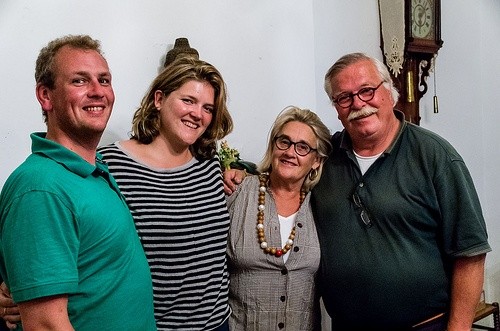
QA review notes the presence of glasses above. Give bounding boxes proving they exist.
[350,189,375,231]
[333,80,385,109]
[273,134,319,157]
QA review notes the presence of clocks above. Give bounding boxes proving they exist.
[377,0,445,126]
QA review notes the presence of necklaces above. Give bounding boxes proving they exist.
[257,175,306,258]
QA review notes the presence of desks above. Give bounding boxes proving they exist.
[471,301,500,331]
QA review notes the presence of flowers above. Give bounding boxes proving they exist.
[219,140,240,171]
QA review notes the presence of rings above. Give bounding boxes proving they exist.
[4,307,7,314]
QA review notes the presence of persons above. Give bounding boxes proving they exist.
[225,104,332,331]
[0,57,235,331]
[222,51,492,331]
[0,34,158,331]
